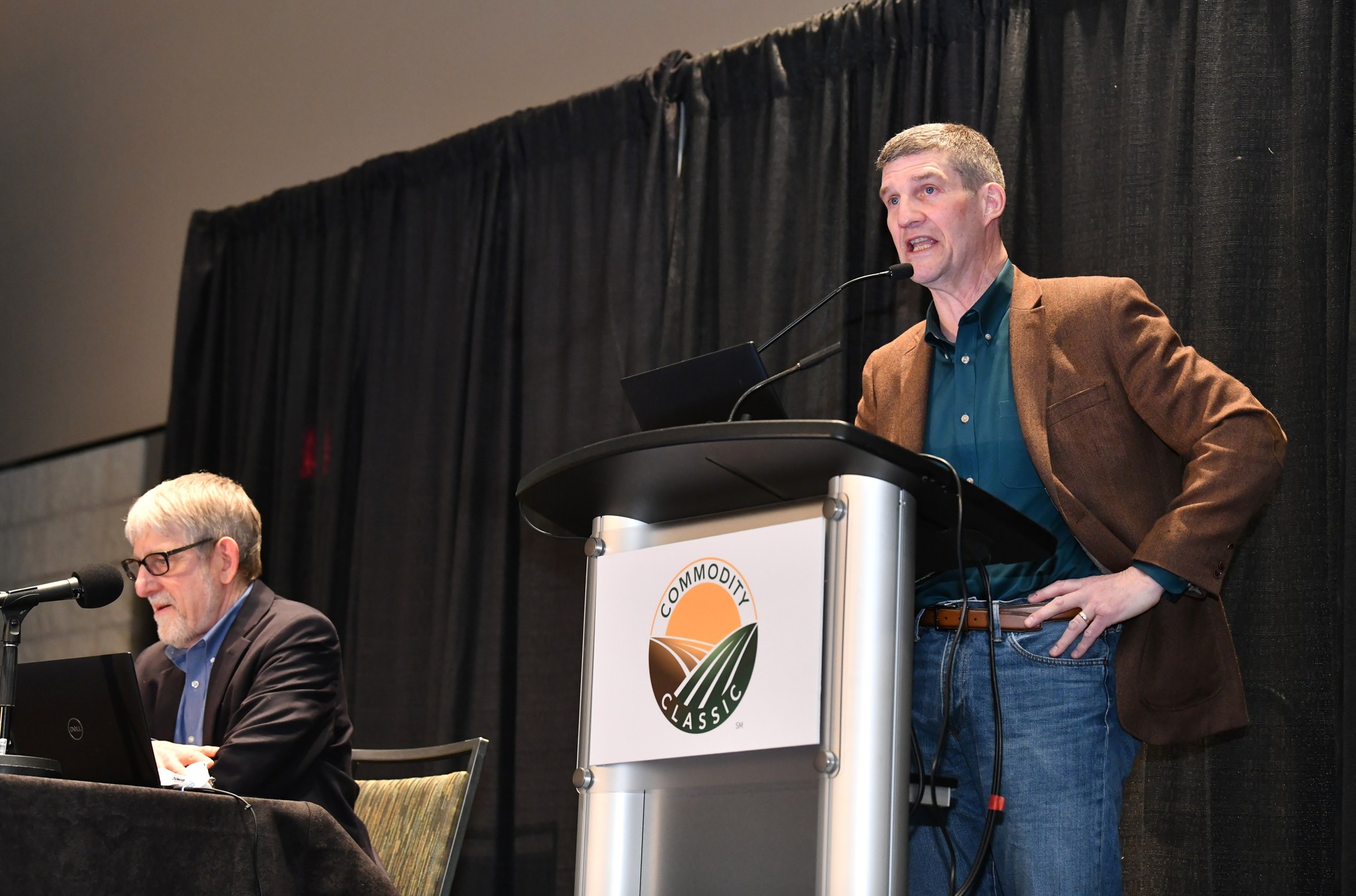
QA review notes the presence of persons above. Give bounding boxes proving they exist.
[850,117,1293,896]
[123,472,360,823]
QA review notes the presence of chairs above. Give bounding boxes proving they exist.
[343,736,491,896]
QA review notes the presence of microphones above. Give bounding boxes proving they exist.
[0,562,124,626]
[726,340,843,422]
[173,786,193,792]
[753,263,914,355]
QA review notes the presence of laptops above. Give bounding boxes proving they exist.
[6,653,162,788]
[623,342,789,429]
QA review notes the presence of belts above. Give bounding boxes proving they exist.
[920,603,1082,632]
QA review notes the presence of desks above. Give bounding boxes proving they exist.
[0,767,390,896]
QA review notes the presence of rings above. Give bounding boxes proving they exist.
[1078,612,1091,624]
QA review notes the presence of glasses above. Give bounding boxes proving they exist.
[120,538,212,581]
